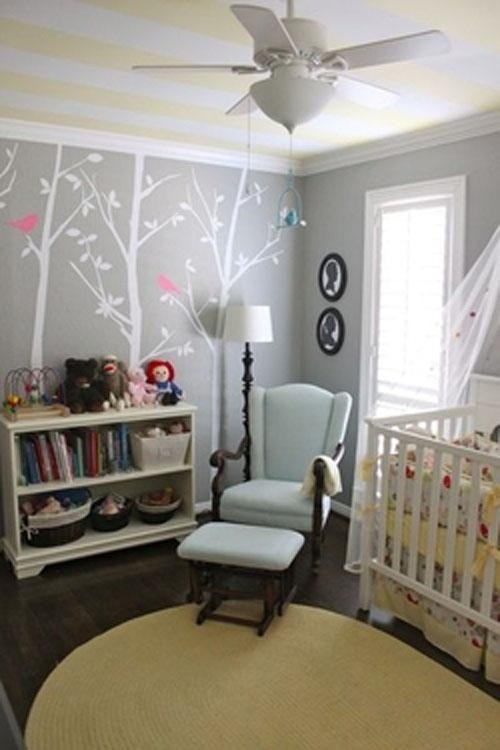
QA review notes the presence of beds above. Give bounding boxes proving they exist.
[357,372,500,690]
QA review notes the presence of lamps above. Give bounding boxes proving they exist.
[249,66,335,231]
[221,305,275,480]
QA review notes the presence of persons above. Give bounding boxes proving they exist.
[321,315,336,346]
[324,262,338,294]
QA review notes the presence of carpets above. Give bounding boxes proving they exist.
[22,595,499,750]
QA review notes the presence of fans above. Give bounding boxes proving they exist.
[129,1,453,119]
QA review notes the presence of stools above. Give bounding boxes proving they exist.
[176,520,306,636]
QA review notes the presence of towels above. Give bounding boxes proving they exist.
[299,454,343,500]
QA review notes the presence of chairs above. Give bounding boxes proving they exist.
[208,382,353,577]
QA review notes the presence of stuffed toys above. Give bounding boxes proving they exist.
[145,419,183,437]
[147,359,186,405]
[100,353,132,410]
[126,366,157,408]
[57,358,109,412]
[22,491,84,515]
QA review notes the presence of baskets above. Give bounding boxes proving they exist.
[90,496,133,531]
[137,488,180,522]
[18,488,91,547]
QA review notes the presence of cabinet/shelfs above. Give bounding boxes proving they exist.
[1,401,197,579]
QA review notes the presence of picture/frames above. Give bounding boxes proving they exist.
[316,306,344,356]
[318,253,348,303]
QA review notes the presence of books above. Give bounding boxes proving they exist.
[15,423,129,485]
